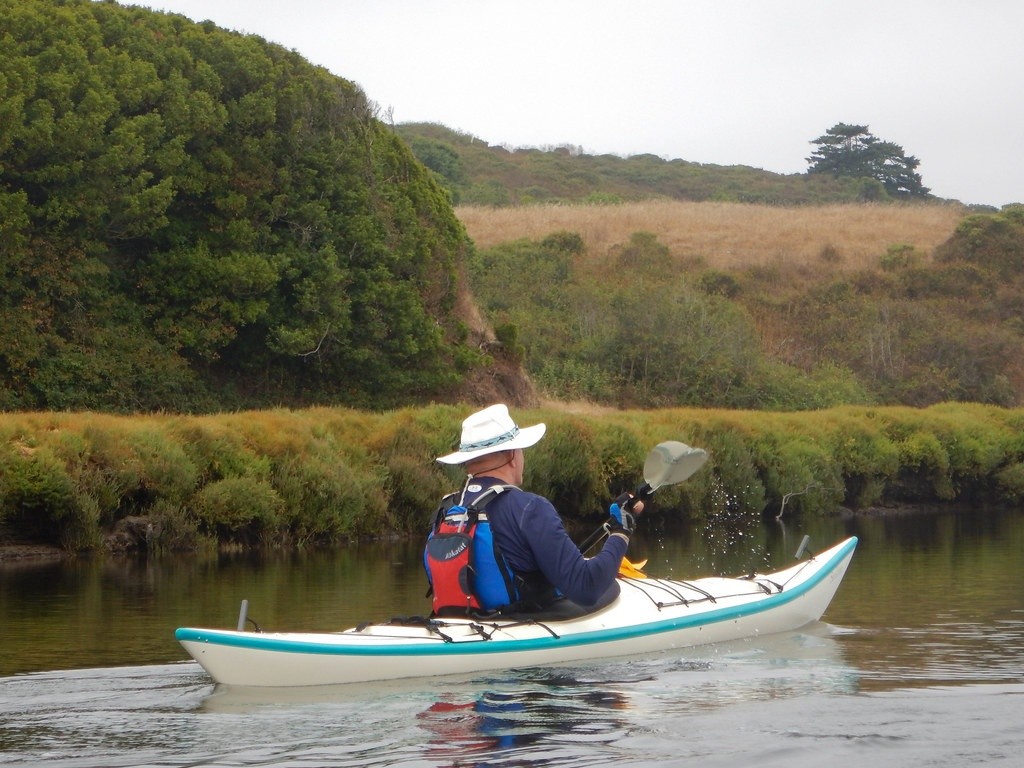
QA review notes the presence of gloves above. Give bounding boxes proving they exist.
[604,492,645,539]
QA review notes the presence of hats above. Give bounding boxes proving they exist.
[436,403,546,465]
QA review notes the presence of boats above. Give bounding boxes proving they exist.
[174,536,859,686]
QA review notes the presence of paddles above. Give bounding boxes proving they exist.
[578,440,708,555]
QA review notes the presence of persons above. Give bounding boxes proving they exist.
[423,403,639,616]
